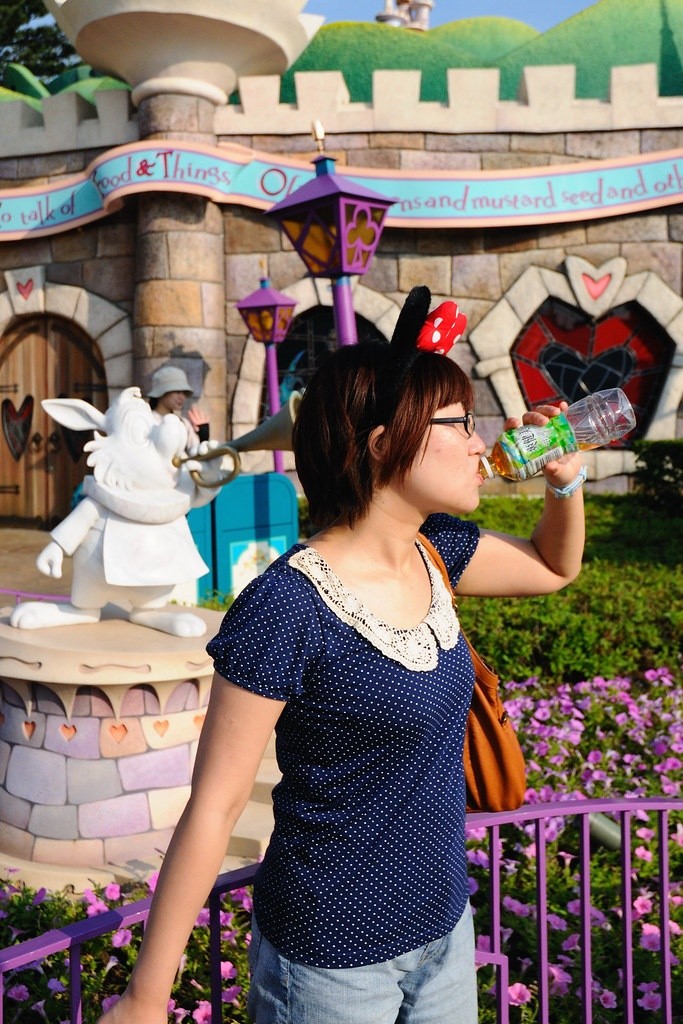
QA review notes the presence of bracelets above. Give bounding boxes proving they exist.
[544,467,586,499]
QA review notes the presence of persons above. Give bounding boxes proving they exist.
[98,343,586,1024]
[147,365,201,456]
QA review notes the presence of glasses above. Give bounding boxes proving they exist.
[428,412,475,436]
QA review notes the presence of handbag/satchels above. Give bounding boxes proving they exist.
[416,532,527,812]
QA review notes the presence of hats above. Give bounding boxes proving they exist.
[146,367,193,399]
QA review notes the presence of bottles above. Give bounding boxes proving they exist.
[478,386,637,479]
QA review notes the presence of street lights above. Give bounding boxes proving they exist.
[233,258,303,475]
[265,118,402,344]
